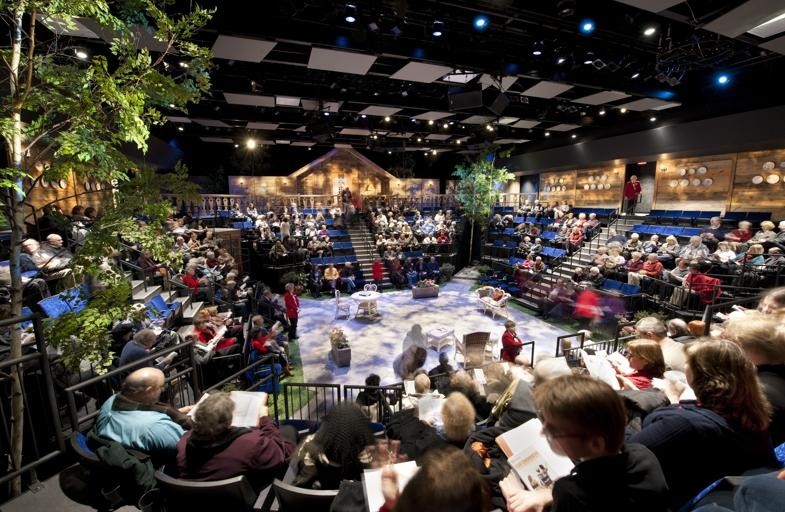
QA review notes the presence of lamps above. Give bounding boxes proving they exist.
[431,17,445,38]
[342,0,359,25]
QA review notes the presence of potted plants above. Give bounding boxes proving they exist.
[279,270,311,296]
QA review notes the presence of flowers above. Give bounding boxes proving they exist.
[328,326,349,349]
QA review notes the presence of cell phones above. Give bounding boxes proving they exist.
[773,441,785,466]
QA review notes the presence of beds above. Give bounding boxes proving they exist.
[425,326,455,353]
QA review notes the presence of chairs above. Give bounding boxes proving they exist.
[593,209,773,315]
[247,344,282,393]
[452,331,491,371]
[0,238,181,403]
[217,207,366,290]
[402,204,615,298]
[333,281,382,321]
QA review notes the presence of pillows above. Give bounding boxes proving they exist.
[478,289,488,299]
[490,289,503,301]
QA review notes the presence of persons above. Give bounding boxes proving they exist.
[19,189,785,512]
[623,175,642,214]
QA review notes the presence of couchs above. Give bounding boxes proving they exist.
[473,284,511,321]
